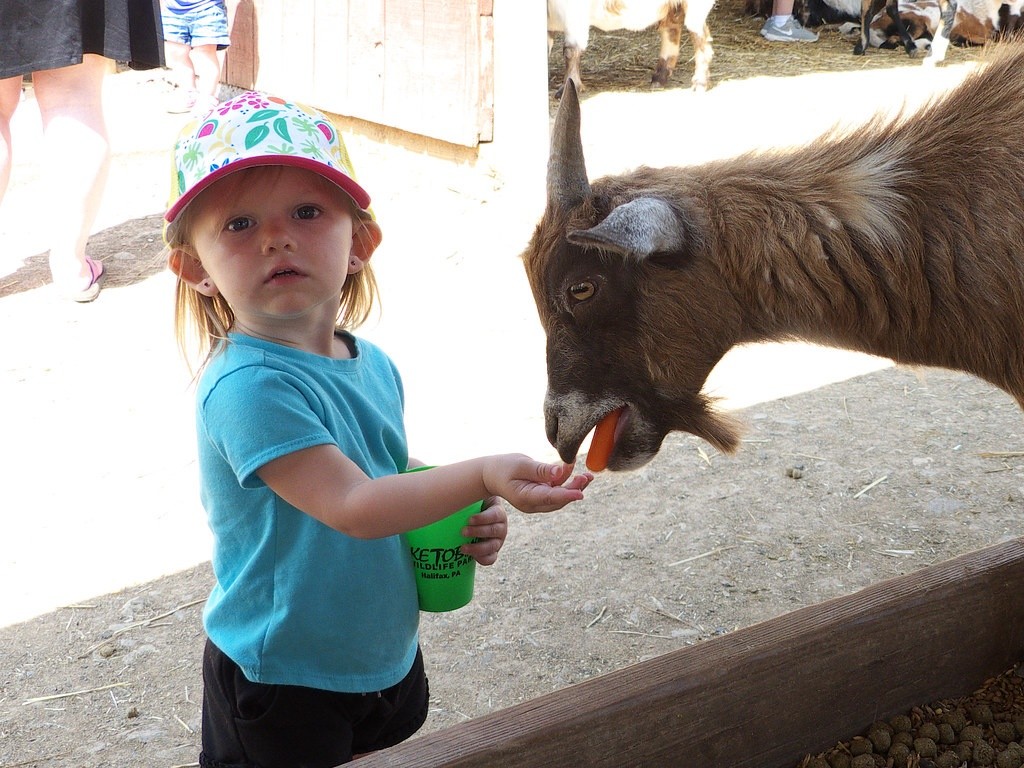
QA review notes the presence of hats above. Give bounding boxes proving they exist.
[165,95,369,231]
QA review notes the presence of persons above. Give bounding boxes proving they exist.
[160,92,593,766]
[0,1,166,303]
[165,1,233,114]
[760,0,820,43]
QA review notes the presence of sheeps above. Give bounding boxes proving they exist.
[519,28,1024,472]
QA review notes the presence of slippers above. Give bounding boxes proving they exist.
[69,256,107,302]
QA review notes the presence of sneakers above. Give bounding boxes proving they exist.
[760,17,772,37]
[764,16,818,43]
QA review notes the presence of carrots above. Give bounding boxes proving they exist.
[586,408,622,472]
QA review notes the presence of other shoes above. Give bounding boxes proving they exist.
[194,96,221,122]
[167,99,194,115]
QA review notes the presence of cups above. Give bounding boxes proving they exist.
[401,465,484,613]
